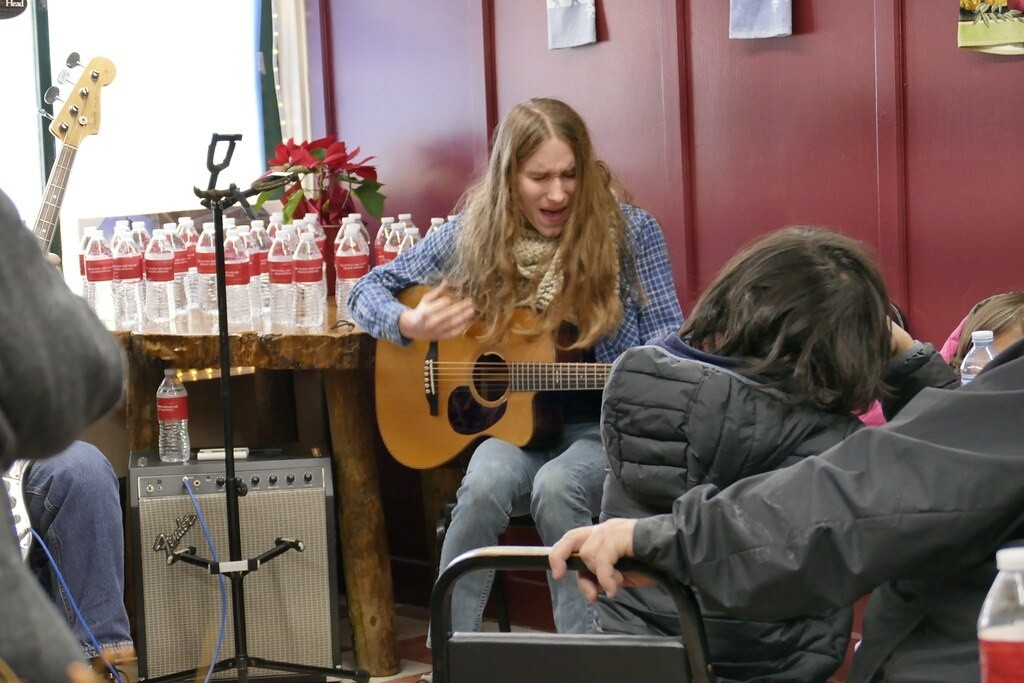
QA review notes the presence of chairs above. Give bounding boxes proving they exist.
[433,488,714,683]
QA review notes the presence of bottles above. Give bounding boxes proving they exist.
[155,368,191,463]
[977,547,1024,683]
[960,330,999,388]
[79,211,458,336]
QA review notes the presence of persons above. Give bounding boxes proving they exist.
[849,292,1024,430]
[547,339,1024,683]
[348,97,684,682]
[599,221,963,683]
[0,187,142,683]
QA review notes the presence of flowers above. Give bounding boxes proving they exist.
[267,133,388,226]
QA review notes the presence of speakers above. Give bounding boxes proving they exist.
[128,441,340,683]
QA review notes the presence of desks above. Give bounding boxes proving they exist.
[111,297,414,678]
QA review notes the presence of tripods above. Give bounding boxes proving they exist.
[133,186,370,683]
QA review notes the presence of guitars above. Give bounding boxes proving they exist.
[0,48,121,562]
[368,279,617,476]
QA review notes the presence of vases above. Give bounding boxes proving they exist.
[322,225,341,293]
